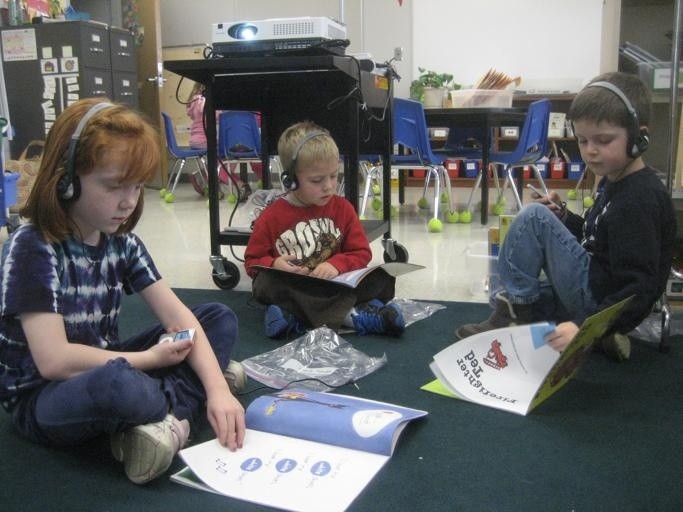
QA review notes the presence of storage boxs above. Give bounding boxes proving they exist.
[450,90,513,108]
[636,60,683,94]
[413,158,585,180]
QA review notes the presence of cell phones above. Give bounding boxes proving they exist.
[156,328,196,343]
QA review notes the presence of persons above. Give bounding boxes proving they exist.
[243,121,405,339]
[215,110,273,203]
[453,72,677,361]
[0,97,252,483]
[184,81,222,194]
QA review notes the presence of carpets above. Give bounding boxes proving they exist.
[0,286,683,511]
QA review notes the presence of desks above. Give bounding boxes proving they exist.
[396,106,527,225]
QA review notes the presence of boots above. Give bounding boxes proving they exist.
[453,293,535,342]
[600,330,632,364]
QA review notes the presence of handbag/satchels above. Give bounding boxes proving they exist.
[4,139,46,214]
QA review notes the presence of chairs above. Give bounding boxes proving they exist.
[359,99,460,233]
[218,111,263,203]
[417,122,503,222]
[459,98,550,224]
[159,112,208,203]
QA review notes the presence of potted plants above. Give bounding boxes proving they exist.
[408,66,458,108]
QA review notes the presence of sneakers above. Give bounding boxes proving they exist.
[238,182,251,203]
[108,413,191,488]
[348,298,409,335]
[216,359,249,391]
[261,304,304,337]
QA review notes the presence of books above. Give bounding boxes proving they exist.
[247,261,426,288]
[420,292,636,414]
[616,39,673,74]
[170,385,432,511]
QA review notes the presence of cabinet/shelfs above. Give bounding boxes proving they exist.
[601,0,683,195]
[2,20,138,163]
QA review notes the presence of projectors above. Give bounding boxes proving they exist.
[210,15,348,55]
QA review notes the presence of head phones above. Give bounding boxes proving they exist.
[281,132,329,190]
[56,102,115,202]
[570,80,651,158]
